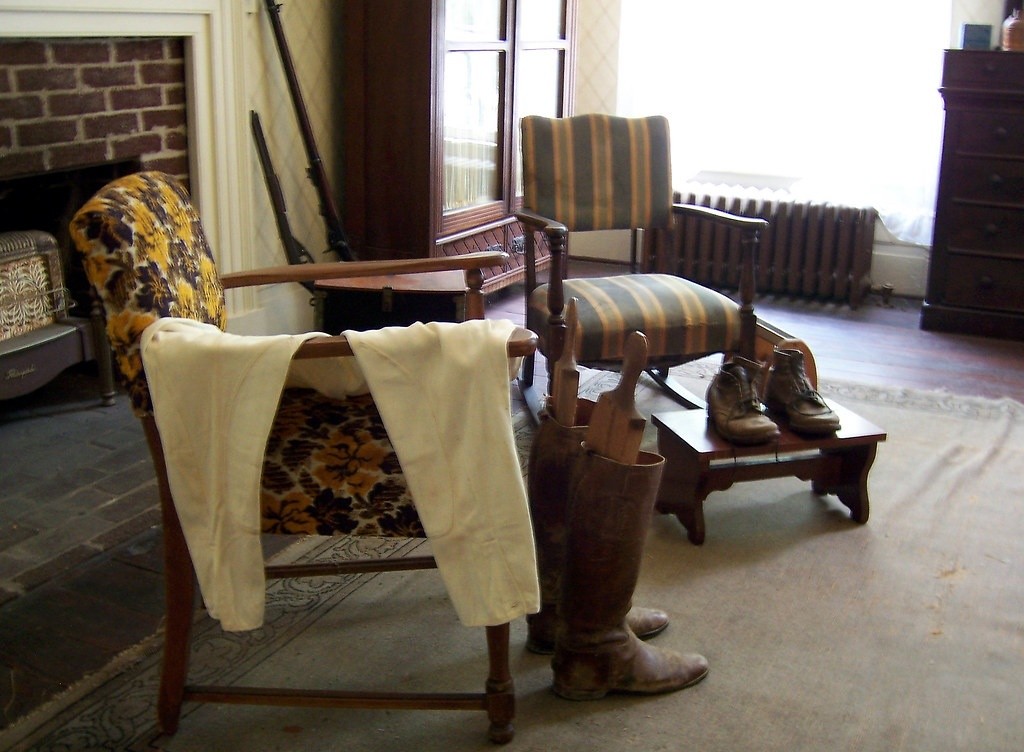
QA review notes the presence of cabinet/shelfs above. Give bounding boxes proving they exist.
[343,0,577,296]
[920,49,1024,343]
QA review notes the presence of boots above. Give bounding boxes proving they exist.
[528,398,670,655]
[707,356,781,443]
[551,441,709,700]
[761,349,842,434]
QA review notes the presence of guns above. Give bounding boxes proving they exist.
[263,0,359,263]
[248,107,320,297]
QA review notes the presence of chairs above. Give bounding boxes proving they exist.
[0,172,539,752]
[514,113,771,425]
[0,230,118,407]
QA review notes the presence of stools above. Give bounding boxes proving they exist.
[651,398,887,545]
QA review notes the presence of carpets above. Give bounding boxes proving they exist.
[145,362,1024,752]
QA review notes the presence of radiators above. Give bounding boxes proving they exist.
[641,192,878,311]
